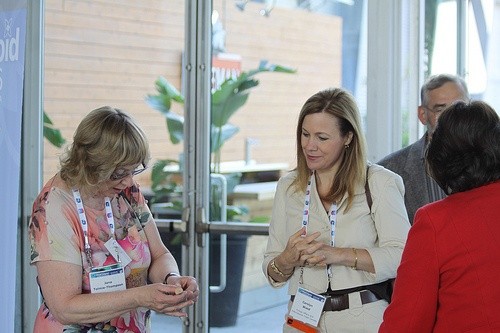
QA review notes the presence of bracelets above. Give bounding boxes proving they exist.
[163,273,179,284]
[351,247,359,270]
[271,258,294,279]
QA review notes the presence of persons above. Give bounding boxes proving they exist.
[29,106,200,333]
[373,74,469,226]
[378,101,500,333]
[262,86,413,333]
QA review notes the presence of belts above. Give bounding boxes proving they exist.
[290,289,380,312]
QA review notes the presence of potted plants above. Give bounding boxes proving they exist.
[144,61,297,328]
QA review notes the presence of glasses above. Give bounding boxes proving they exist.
[110,160,147,180]
[424,105,444,118]
